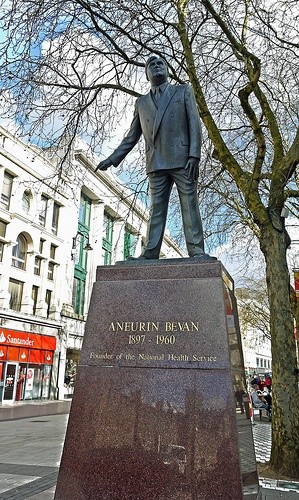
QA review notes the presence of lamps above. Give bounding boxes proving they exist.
[71,231,93,260]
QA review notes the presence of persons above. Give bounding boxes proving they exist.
[251,372,273,421]
[93,56,212,260]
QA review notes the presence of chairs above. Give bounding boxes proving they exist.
[250,395,271,422]
